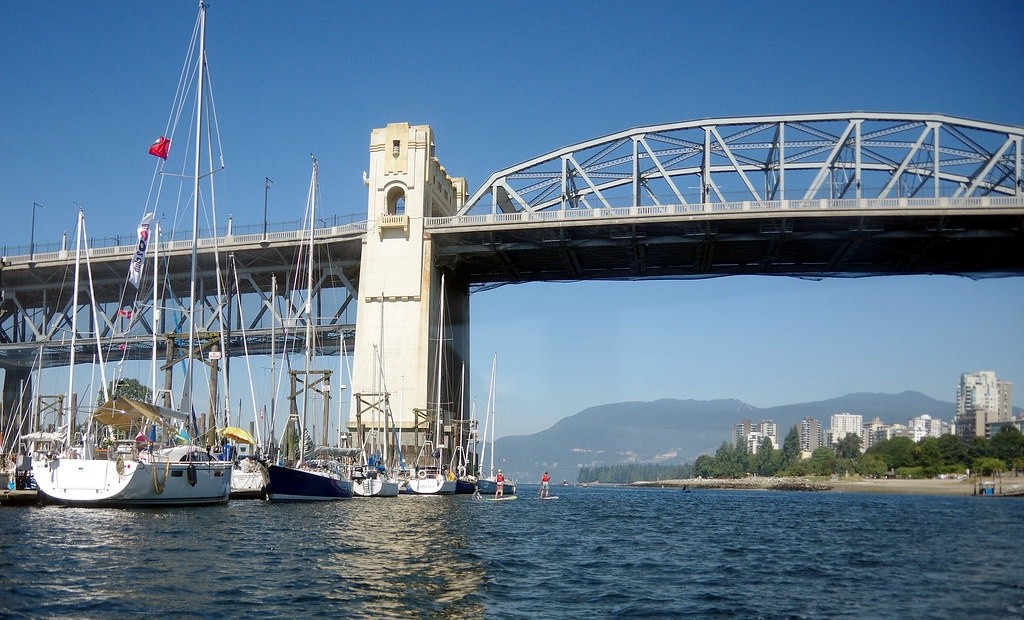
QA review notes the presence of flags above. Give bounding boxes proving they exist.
[149,136,171,160]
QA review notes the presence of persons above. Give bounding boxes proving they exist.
[540,470,550,499]
[144,441,155,454]
[495,469,505,498]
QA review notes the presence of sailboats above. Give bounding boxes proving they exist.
[1,1,518,508]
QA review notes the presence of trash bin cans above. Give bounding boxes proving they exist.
[984,481,995,495]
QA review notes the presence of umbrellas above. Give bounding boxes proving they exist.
[215,427,255,461]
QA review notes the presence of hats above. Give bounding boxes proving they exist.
[545,471,548,474]
[498,469,502,473]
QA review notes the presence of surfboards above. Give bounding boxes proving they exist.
[534,496,558,499]
[486,495,517,501]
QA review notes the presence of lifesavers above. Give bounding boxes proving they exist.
[418,470,427,479]
